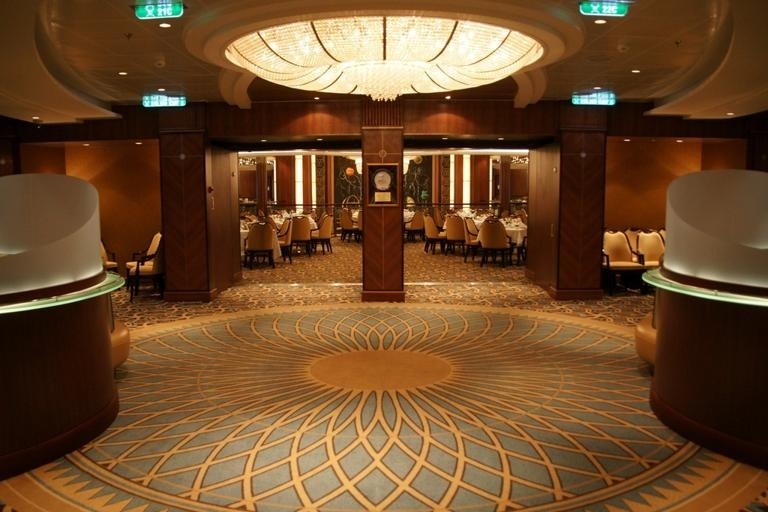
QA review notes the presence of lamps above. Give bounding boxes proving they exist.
[219,9,549,103]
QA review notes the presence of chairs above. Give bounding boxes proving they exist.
[463,217,488,264]
[126,231,162,291]
[404,212,424,241]
[659,229,667,242]
[423,215,445,255]
[445,214,466,256]
[433,207,444,232]
[603,230,643,296]
[101,242,121,292]
[341,212,359,243]
[128,244,165,303]
[516,236,528,266]
[625,230,641,262]
[478,217,512,268]
[277,220,293,265]
[290,216,312,257]
[357,211,364,243]
[244,222,276,271]
[309,216,333,256]
[637,232,667,296]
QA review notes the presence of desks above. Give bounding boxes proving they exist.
[1,173,125,483]
[641,170,768,472]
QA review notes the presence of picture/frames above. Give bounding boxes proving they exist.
[366,163,400,208]
